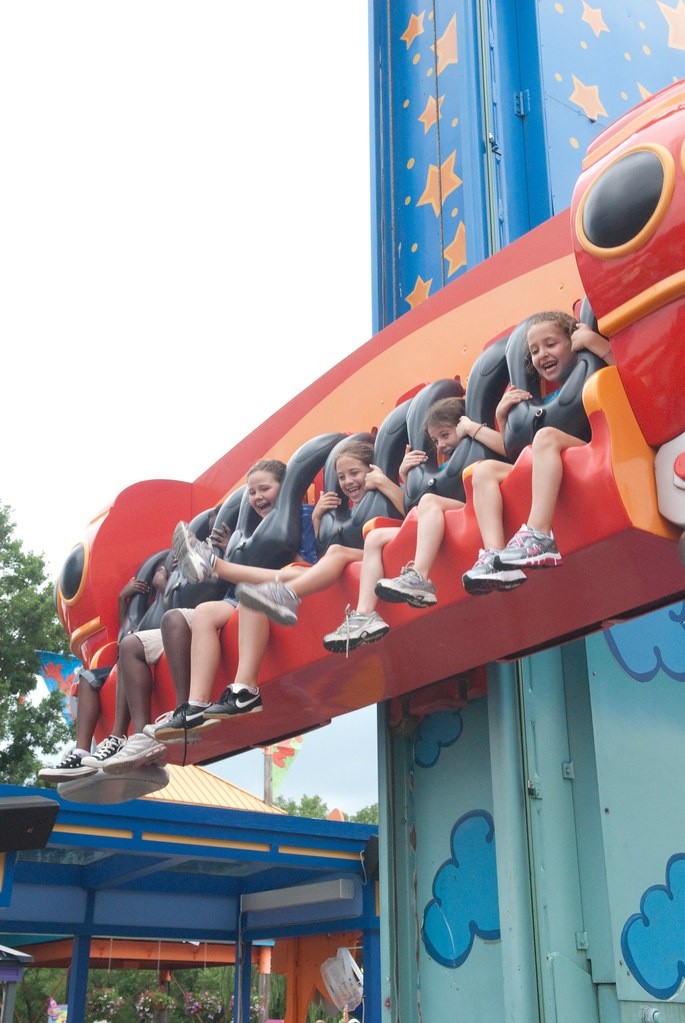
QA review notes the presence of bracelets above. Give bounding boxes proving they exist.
[472,424,487,440]
[599,349,611,358]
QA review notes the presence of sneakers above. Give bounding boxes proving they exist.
[101,732,168,775]
[81,735,126,769]
[203,686,263,719]
[321,603,389,658]
[234,576,300,625]
[172,520,219,584]
[143,710,182,743]
[155,702,221,767]
[492,523,563,570]
[374,560,437,609]
[462,547,526,595]
[38,753,99,783]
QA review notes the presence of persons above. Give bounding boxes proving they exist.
[101,504,233,774]
[37,566,168,783]
[462,311,617,596]
[154,459,317,767]
[322,396,506,659]
[173,440,404,627]
